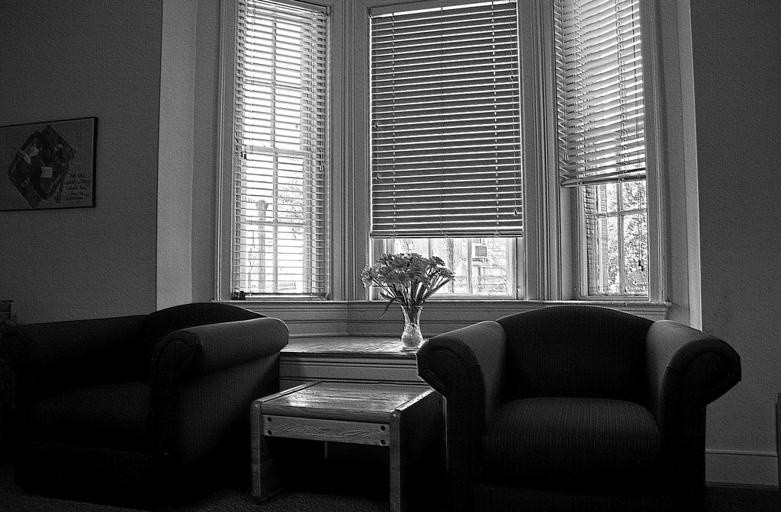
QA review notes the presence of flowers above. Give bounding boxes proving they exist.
[361,253,455,324]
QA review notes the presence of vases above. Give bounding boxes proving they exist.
[400,306,423,350]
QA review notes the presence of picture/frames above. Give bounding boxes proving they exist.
[0,117,97,212]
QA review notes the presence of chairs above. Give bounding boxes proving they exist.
[417,305,741,511]
[11,302,290,511]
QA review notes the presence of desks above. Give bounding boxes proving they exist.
[249,380,444,511]
